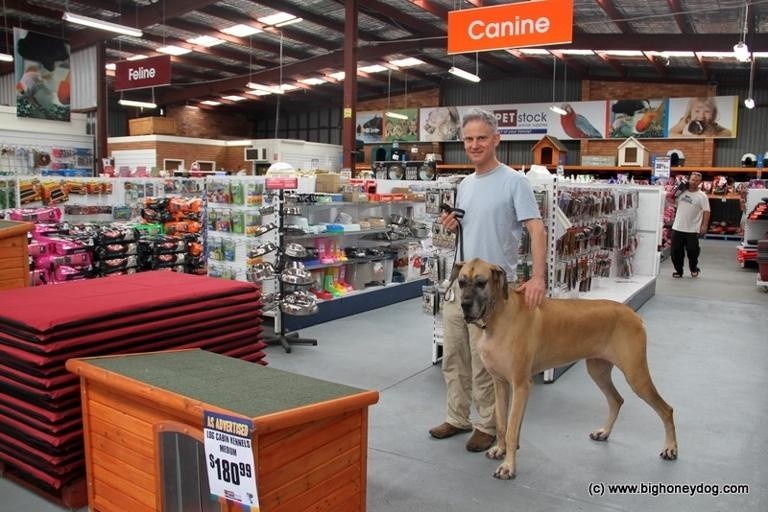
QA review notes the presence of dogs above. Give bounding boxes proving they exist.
[449,257,677,480]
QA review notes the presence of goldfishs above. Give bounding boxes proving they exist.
[636,98,663,132]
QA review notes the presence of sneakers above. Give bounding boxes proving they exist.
[467,428,496,452]
[673,270,681,278]
[691,271,698,277]
[429,422,472,438]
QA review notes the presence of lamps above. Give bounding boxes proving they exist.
[118,35,158,110]
[385,68,408,121]
[734,1,749,63]
[448,1,480,84]
[550,55,569,115]
[62,1,144,39]
[743,51,758,110]
[246,34,285,95]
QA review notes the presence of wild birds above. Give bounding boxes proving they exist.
[559,102,602,138]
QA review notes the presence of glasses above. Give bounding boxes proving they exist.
[692,172,701,177]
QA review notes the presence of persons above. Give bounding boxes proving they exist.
[667,96,732,138]
[384,115,419,138]
[668,170,711,278]
[17,66,53,100]
[423,105,549,453]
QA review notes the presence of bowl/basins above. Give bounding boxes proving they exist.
[246,194,320,317]
[388,213,408,241]
